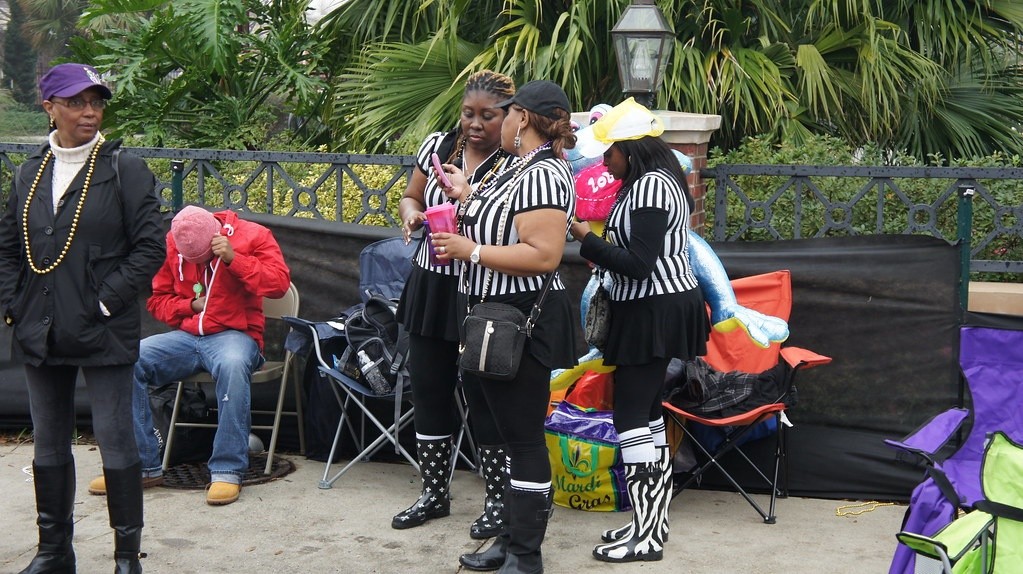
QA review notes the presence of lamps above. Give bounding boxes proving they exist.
[609,0,679,109]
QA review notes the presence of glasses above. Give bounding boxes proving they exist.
[48,98,107,111]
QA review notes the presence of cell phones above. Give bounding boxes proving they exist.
[432,152,453,188]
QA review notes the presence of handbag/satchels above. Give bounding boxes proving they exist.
[585,285,611,355]
[459,302,526,380]
[543,398,633,512]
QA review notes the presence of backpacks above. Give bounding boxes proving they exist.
[338,288,411,393]
[148,382,211,465]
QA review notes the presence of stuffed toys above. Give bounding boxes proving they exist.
[548,103,789,391]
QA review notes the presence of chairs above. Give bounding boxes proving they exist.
[160,276,300,477]
[661,270,831,525]
[282,235,483,493]
[885,312,1023,574]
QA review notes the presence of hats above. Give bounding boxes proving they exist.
[38,63,112,101]
[494,80,571,121]
[573,96,664,159]
[171,205,221,264]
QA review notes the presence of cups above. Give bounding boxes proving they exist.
[424,202,460,265]
[423,220,452,267]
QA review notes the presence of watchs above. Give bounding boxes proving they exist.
[470,245,481,264]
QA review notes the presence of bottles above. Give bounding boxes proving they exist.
[357,350,392,395]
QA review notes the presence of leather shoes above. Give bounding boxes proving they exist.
[204,482,242,505]
[88,474,163,495]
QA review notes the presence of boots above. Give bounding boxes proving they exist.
[496,484,554,574]
[102,460,147,574]
[602,445,673,542]
[592,459,665,562]
[18,458,76,574]
[458,472,510,570]
[470,444,506,539]
[391,435,452,530]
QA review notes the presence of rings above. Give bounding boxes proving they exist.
[402,228,404,231]
[440,247,445,254]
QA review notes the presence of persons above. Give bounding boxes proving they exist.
[391,71,515,539]
[429,81,574,574]
[570,96,710,563]
[88,206,289,505]
[0,64,167,574]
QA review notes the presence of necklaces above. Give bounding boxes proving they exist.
[458,138,551,303]
[192,264,207,299]
[22,138,103,274]
[836,501,895,516]
[602,186,626,239]
